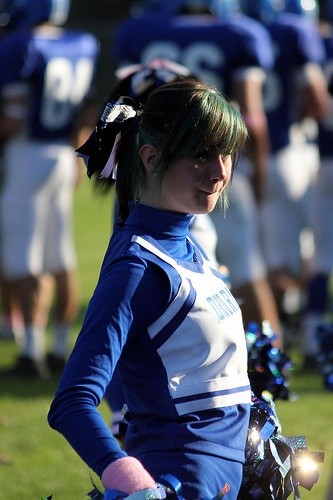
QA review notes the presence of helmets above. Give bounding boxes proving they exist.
[7,0,70,27]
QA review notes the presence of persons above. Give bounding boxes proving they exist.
[0,0,333,399]
[47,59,254,500]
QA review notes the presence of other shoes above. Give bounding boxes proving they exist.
[46,354,64,373]
[15,355,38,380]
